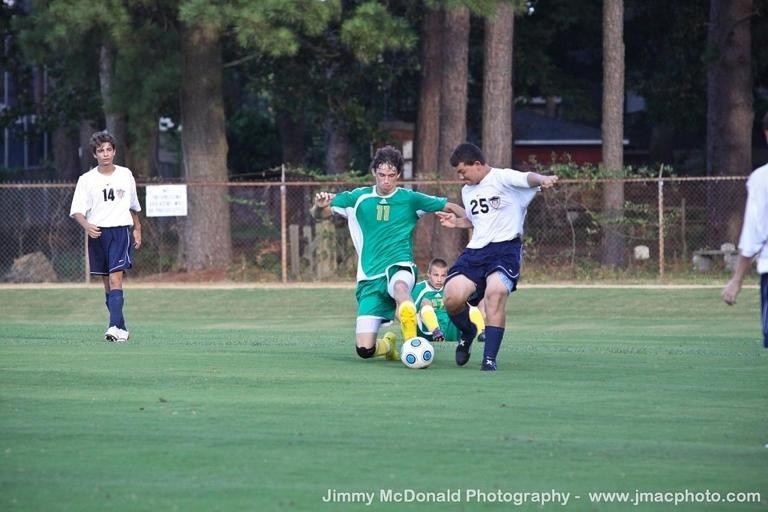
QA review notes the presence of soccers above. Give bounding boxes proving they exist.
[399,336,434,369]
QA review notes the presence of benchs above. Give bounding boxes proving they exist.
[693,251,739,274]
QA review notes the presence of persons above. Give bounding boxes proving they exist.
[310,144,474,362]
[434,142,559,372]
[721,113,768,349]
[69,131,142,342]
[410,258,487,342]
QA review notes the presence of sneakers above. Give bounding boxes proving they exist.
[454,326,477,367]
[116,329,129,342]
[433,335,444,342]
[398,301,417,341]
[383,334,400,363]
[104,326,119,341]
[480,355,497,371]
